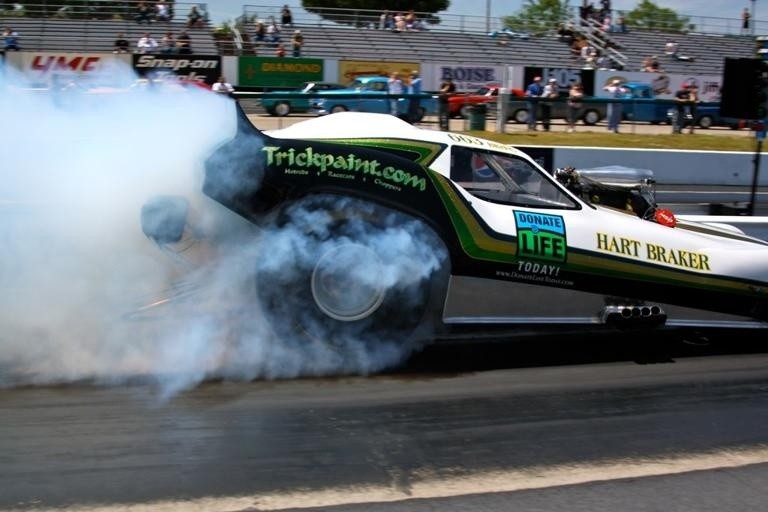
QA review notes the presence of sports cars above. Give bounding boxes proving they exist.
[130,101,768,377]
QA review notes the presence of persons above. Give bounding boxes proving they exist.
[527,77,557,133]
[740,7,751,34]
[602,79,630,134]
[566,84,582,134]
[113,0,303,56]
[674,84,700,133]
[557,0,693,72]
[210,75,235,97]
[2,26,20,51]
[437,76,457,133]
[377,8,421,33]
[388,70,423,125]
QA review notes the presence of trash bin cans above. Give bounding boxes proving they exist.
[463,102,487,132]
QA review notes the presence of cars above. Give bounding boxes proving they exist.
[311,76,438,123]
[505,88,608,123]
[595,83,686,125]
[262,83,347,114]
[692,101,765,131]
[448,85,528,118]
